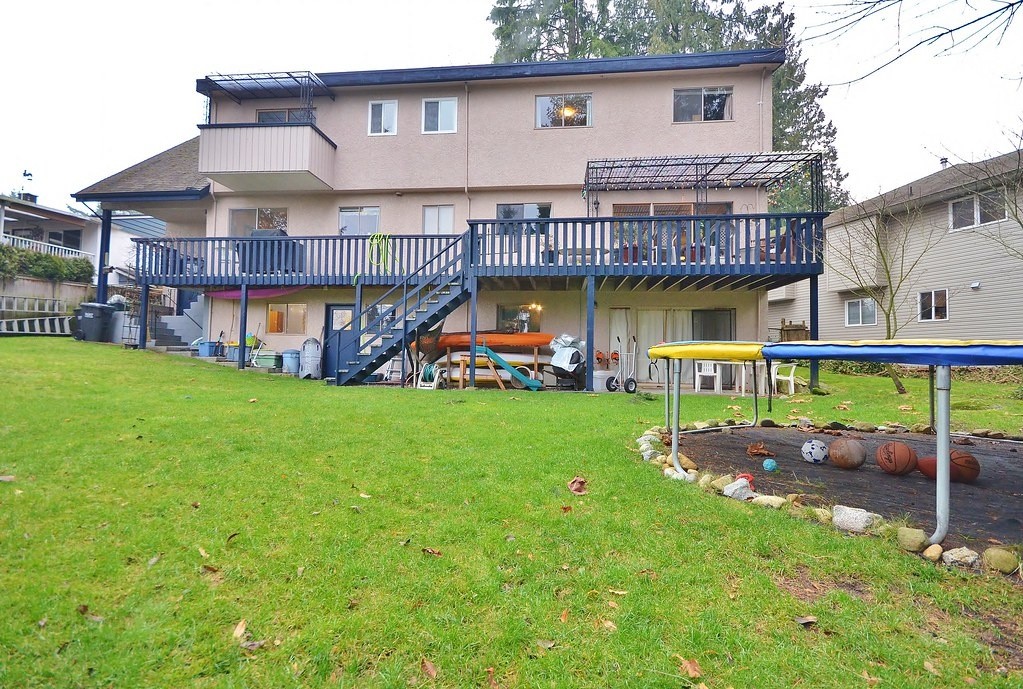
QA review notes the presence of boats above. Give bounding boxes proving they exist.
[411,331,556,384]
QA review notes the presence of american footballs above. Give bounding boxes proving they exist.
[917,456,961,481]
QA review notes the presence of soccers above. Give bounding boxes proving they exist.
[800,438,829,464]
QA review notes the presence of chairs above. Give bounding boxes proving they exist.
[751,362,799,396]
[652,221,677,265]
[702,220,735,262]
[752,220,799,262]
[695,359,717,392]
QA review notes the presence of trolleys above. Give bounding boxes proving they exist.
[606,335,637,393]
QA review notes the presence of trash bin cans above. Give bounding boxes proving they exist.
[282,349,300,372]
[299,337,323,380]
[72,301,114,342]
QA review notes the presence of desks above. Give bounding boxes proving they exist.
[559,249,610,263]
[712,361,764,396]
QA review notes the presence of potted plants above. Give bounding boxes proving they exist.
[540,232,562,264]
[612,222,642,263]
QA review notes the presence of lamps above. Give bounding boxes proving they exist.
[594,200,600,210]
[971,282,982,289]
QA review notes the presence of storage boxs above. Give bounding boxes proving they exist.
[692,114,702,121]
[225,345,252,362]
[198,341,223,356]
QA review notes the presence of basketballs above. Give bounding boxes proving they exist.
[876,440,919,476]
[829,438,867,469]
[949,448,981,482]
